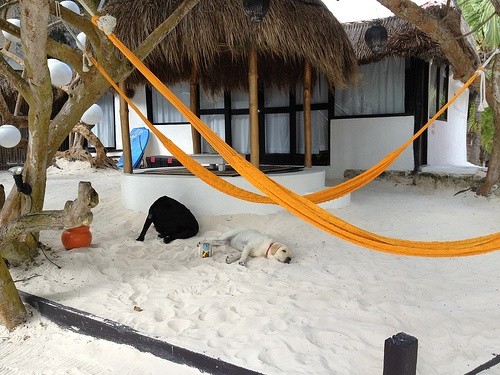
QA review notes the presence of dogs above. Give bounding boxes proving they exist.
[208,226,292,266]
[136,196,200,244]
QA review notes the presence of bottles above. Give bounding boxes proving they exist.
[61,227,94,249]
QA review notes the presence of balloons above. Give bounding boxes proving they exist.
[0,124,22,148]
[81,103,104,125]
[2,17,23,43]
[76,31,87,51]
[60,0,82,15]
[45,57,75,88]
